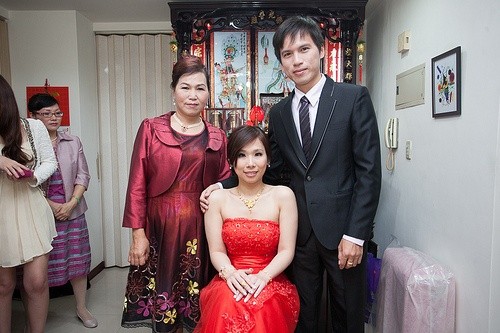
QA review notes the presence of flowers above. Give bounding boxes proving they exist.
[246,106,264,126]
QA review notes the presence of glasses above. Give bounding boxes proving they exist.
[34,111,64,117]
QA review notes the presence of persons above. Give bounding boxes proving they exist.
[19,93,98,333]
[0,74,59,333]
[121,56,231,333]
[193,125,301,333]
[199,17,382,333]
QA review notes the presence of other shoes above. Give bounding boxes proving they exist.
[76,309,98,328]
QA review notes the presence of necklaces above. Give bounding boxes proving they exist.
[236,186,265,214]
[173,113,202,133]
[51,136,57,141]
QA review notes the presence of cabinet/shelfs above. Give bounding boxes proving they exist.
[168,0,368,138]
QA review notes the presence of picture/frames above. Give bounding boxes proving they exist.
[431,46,461,118]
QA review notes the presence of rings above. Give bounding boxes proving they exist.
[347,261,353,265]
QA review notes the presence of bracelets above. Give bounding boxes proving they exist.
[219,265,235,278]
[263,270,272,282]
[72,195,80,205]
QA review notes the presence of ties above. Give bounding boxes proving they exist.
[300,96,312,167]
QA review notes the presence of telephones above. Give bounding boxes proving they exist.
[385,118,398,148]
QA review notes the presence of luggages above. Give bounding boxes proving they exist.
[376,247,455,333]
